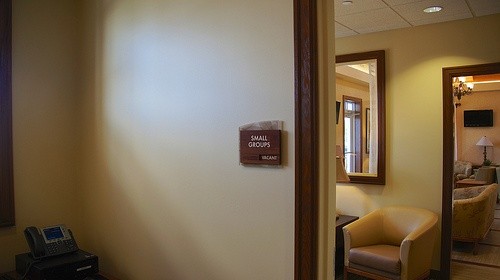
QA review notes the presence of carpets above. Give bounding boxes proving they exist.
[452,204,500,267]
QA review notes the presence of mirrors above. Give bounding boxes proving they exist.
[335,49,386,186]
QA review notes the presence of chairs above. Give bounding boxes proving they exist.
[343,205,440,280]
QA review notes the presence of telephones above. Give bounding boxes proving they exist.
[24,224,78,259]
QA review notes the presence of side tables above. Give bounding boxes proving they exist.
[456,180,487,187]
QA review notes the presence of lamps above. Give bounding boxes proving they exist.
[336,155,350,220]
[475,135,495,162]
[452,76,473,106]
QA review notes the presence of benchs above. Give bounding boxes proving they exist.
[454,183,499,256]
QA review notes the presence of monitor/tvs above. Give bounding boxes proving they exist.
[464,110,493,127]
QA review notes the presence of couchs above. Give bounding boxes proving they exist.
[455,160,472,179]
[472,167,496,182]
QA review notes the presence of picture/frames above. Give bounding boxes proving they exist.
[366,108,373,154]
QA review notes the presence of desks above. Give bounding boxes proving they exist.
[336,215,359,275]
